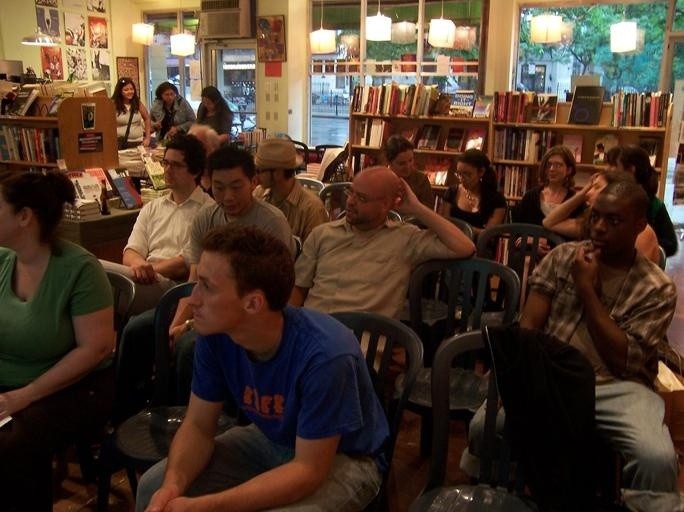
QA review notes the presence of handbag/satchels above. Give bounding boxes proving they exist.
[117,136,129,150]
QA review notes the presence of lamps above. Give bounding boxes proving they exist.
[169,32,197,58]
[608,5,637,52]
[455,26,477,52]
[341,33,360,57]
[427,0,457,49]
[363,0,393,43]
[392,20,415,47]
[309,1,338,56]
[529,8,563,46]
[131,22,156,47]
[21,1,53,47]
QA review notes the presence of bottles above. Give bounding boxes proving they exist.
[100,179,111,215]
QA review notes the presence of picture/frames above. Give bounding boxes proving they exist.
[117,56,140,98]
[255,13,288,63]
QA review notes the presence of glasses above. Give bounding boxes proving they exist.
[454,171,474,179]
[159,159,190,170]
[344,188,391,205]
[546,161,565,169]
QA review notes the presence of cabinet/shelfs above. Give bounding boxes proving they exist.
[315,145,341,162]
[291,141,308,169]
[493,99,675,203]
[1,95,120,178]
[348,94,493,193]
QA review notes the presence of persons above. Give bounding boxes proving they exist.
[1,80,683,511]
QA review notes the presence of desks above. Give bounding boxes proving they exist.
[61,196,142,264]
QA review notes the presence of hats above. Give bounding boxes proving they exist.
[254,139,304,171]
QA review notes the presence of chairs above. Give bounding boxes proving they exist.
[475,223,568,328]
[410,330,596,511]
[328,313,422,510]
[96,271,137,511]
[317,181,352,215]
[337,207,403,224]
[111,282,233,512]
[395,258,522,461]
[295,176,323,196]
[402,213,475,325]
